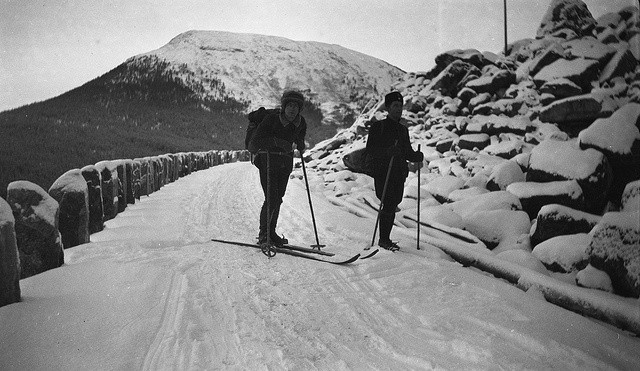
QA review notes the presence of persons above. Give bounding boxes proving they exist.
[248,91,307,247]
[366,92,423,252]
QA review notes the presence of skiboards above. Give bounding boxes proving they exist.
[211,239,379,265]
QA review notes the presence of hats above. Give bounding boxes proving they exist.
[385,92,402,107]
[281,91,304,114]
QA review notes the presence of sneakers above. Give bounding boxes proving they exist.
[379,238,400,252]
[257,236,283,247]
[271,232,288,245]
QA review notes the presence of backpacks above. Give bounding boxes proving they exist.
[245,107,280,163]
